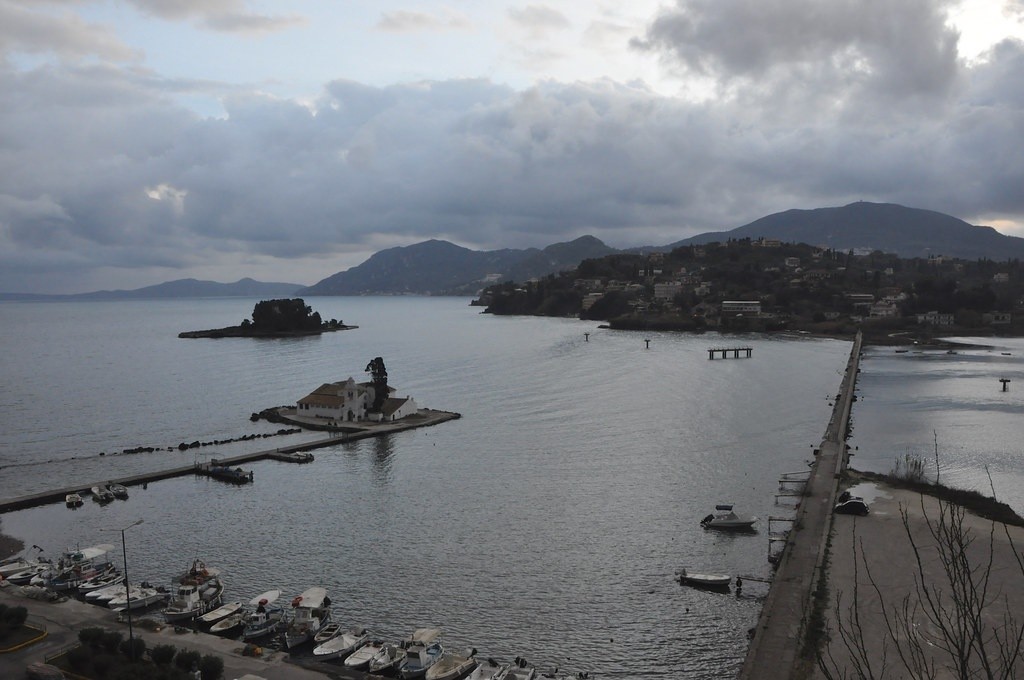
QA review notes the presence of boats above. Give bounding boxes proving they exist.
[91,485,115,501]
[108,580,171,610]
[96,584,140,601]
[464,657,504,680]
[294,451,314,461]
[77,571,126,595]
[344,640,384,666]
[564,672,595,680]
[313,622,341,645]
[83,583,124,603]
[242,590,284,638]
[424,647,478,680]
[497,656,535,680]
[284,587,332,648]
[534,667,564,680]
[312,629,369,662]
[674,568,732,585]
[65,494,82,506]
[400,628,445,680]
[701,504,761,527]
[201,601,242,623]
[110,483,128,496]
[161,546,225,623]
[208,613,245,632]
[369,634,413,675]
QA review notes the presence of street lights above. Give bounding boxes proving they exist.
[99,519,145,665]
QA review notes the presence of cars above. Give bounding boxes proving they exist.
[835,500,870,516]
[1,544,115,590]
